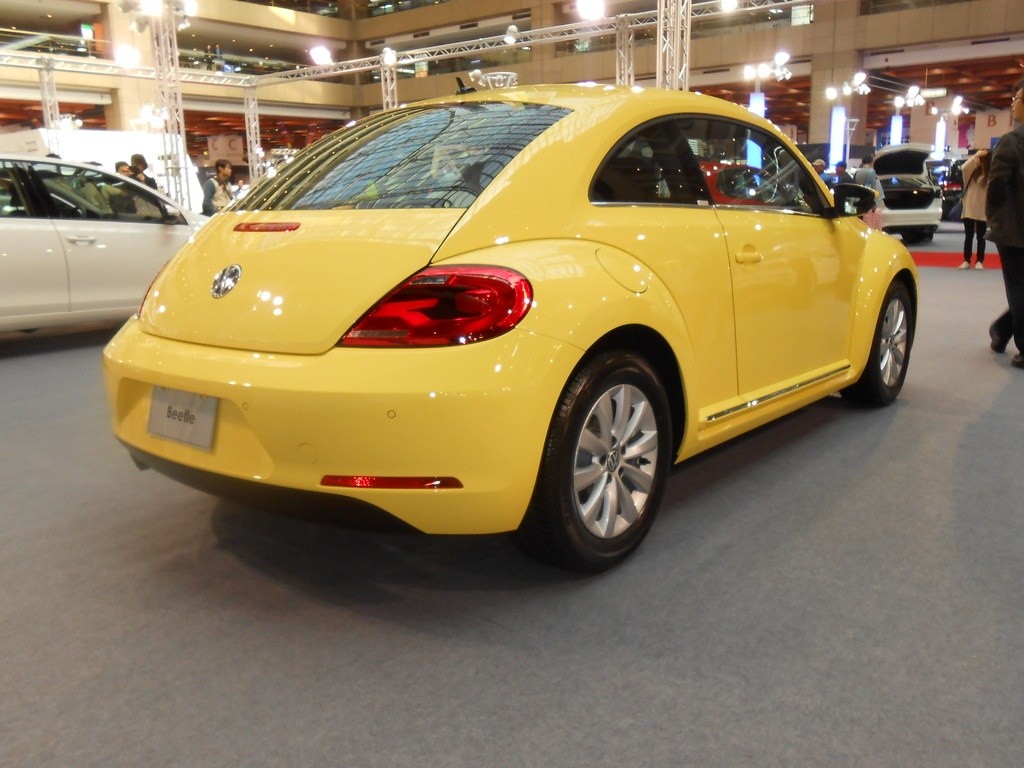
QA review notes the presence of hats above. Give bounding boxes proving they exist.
[812,159,826,168]
[834,162,846,169]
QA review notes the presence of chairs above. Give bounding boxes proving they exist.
[601,154,658,200]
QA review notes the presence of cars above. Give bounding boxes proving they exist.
[865,142,945,242]
[102,76,920,570]
[939,157,967,210]
[697,160,807,210]
[0,155,211,344]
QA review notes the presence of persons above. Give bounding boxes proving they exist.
[812,159,833,188]
[982,80,1024,368]
[46,153,158,213]
[960,148,991,270]
[854,156,879,222]
[202,159,244,217]
[833,161,853,183]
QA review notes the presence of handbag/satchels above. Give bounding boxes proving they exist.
[863,207,882,231]
[948,200,964,221]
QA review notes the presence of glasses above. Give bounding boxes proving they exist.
[1012,96,1024,102]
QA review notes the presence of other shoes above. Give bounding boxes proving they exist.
[974,260,983,270]
[1011,351,1024,367]
[959,261,970,270]
[989,322,1007,353]
